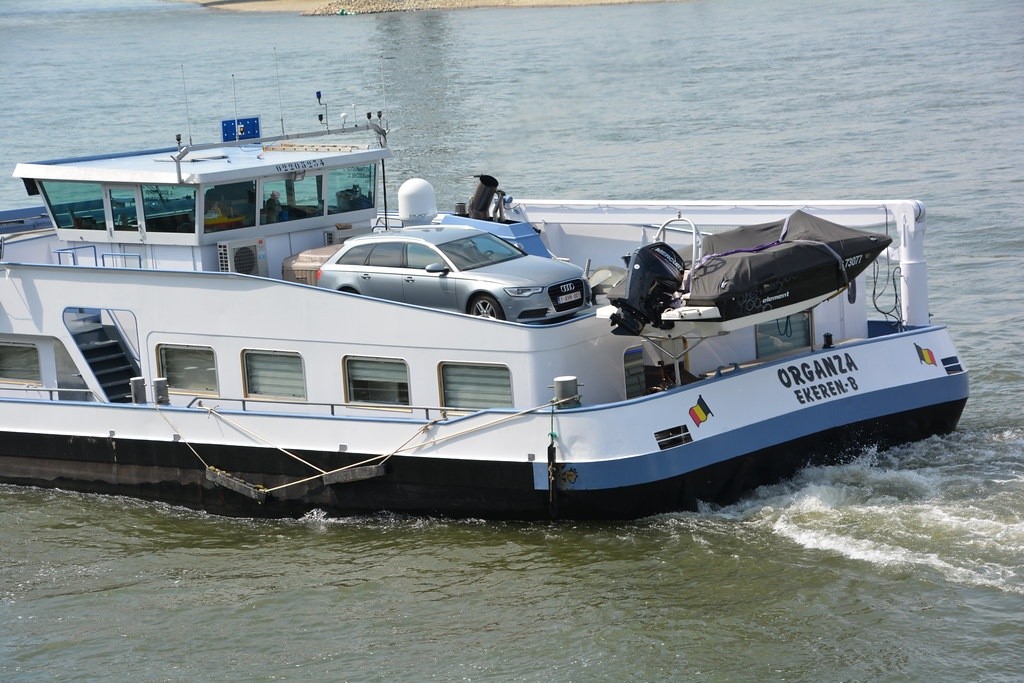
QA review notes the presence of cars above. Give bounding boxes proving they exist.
[316,223,593,319]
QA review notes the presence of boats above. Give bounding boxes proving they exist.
[0,46,973,517]
